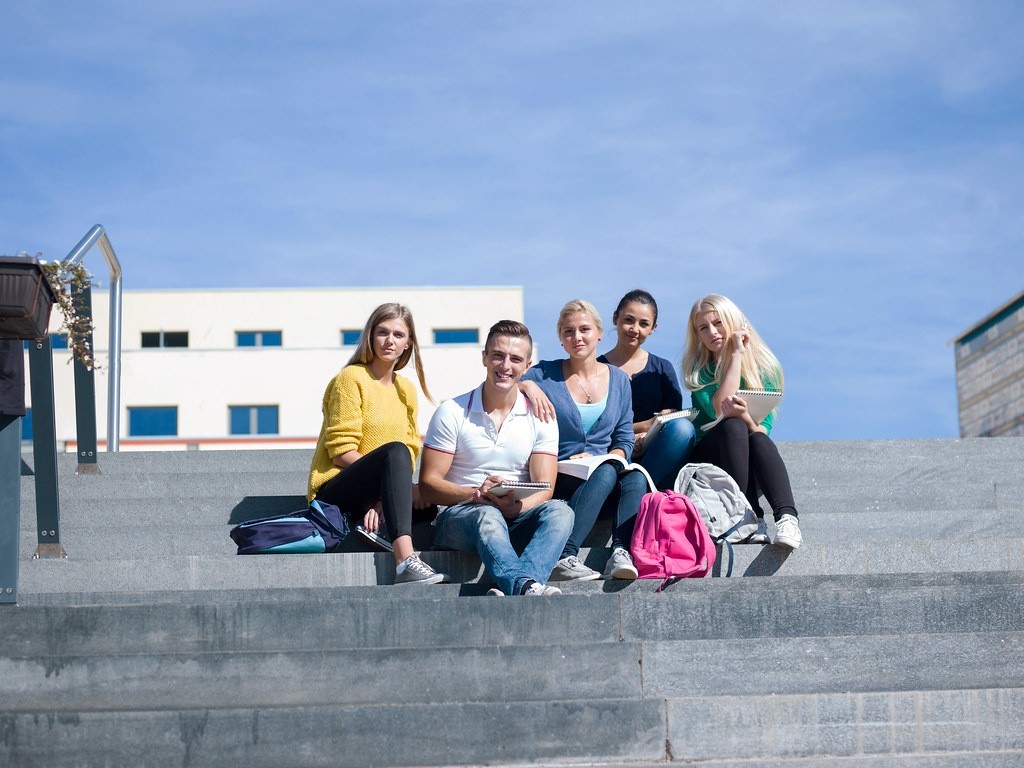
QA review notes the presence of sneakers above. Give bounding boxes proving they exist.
[393,553,451,587]
[547,555,602,581]
[772,512,803,548]
[604,547,638,581]
[355,522,394,552]
[485,588,505,597]
[747,516,770,544]
[524,582,563,596]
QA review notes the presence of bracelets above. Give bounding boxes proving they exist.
[502,500,524,518]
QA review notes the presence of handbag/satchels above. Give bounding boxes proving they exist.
[229,500,350,554]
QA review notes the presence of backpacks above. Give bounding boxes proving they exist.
[675,461,758,545]
[630,489,717,592]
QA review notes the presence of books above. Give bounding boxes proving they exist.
[558,453,659,493]
[631,407,700,459]
[457,481,551,506]
[700,389,784,432]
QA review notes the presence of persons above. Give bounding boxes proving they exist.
[418,320,575,596]
[596,289,697,493]
[307,303,451,586]
[517,299,648,580]
[681,293,801,549]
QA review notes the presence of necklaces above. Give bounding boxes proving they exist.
[567,361,599,404]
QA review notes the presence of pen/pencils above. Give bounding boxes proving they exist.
[653,412,659,415]
[484,471,491,477]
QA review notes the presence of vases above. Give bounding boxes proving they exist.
[0,255,58,350]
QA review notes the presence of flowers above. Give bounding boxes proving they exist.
[17,250,108,377]
